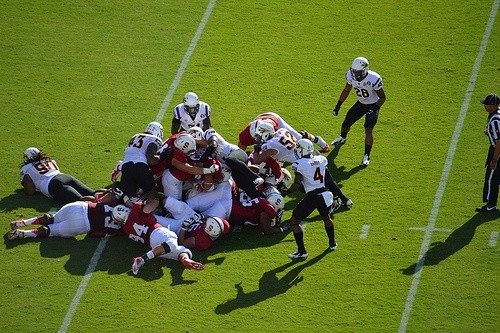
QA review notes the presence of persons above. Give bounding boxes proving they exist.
[3,198,131,241]
[111,187,205,275]
[475,95,500,213]
[94,123,353,251]
[14,147,123,204]
[330,57,386,166]
[237,112,330,153]
[171,92,212,135]
[280,138,338,261]
[118,121,169,215]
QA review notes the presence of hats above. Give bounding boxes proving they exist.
[480,94,500,105]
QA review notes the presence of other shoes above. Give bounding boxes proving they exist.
[475,205,497,214]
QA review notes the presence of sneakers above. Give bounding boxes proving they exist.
[253,177,265,185]
[288,252,308,258]
[362,154,369,166]
[183,259,204,270]
[329,242,337,250]
[339,199,354,206]
[143,199,159,213]
[132,257,144,275]
[331,135,346,145]
[316,136,330,152]
[111,160,123,182]
[8,229,24,240]
[10,219,24,229]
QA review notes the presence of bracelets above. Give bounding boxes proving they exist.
[491,159,498,164]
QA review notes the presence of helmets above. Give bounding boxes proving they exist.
[250,120,262,137]
[23,147,40,163]
[146,121,164,139]
[183,92,199,115]
[187,127,204,140]
[205,216,224,238]
[174,134,196,156]
[281,167,292,189]
[295,139,315,159]
[266,194,285,211]
[112,204,131,224]
[350,57,369,81]
[254,123,275,142]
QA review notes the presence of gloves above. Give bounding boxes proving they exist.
[203,164,220,174]
[254,144,262,154]
[279,221,291,232]
[332,105,340,116]
[181,218,195,231]
[367,108,377,117]
[112,188,129,203]
[193,213,205,222]
